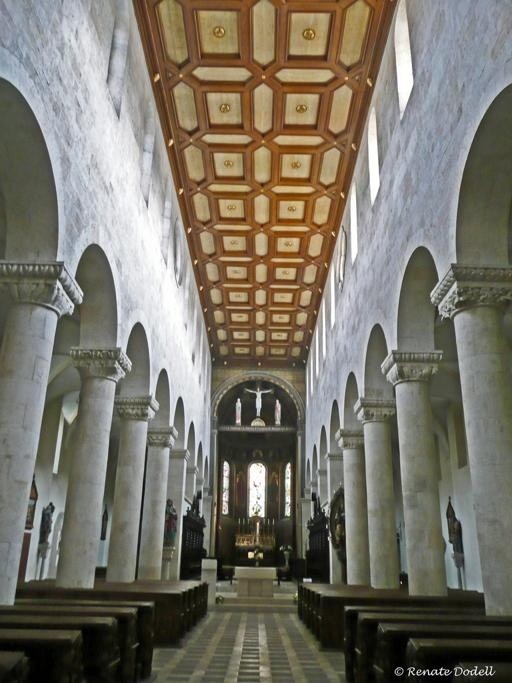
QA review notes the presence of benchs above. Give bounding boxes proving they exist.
[0,577,208,682]
[297,581,511,682]
